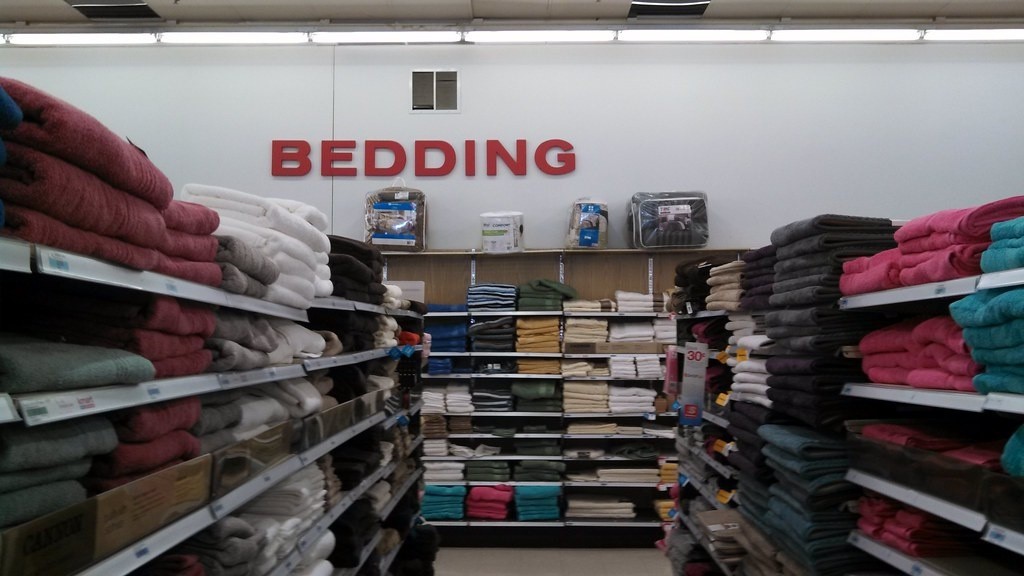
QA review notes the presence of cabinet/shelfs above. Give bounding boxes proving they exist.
[0,240,430,576]
[664,310,740,575]
[377,248,749,526]
[839,268,1024,576]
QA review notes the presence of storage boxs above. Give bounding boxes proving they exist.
[481,212,525,251]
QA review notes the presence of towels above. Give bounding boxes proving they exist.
[416,280,678,523]
[667,194,1024,576]
[0,71,427,576]
